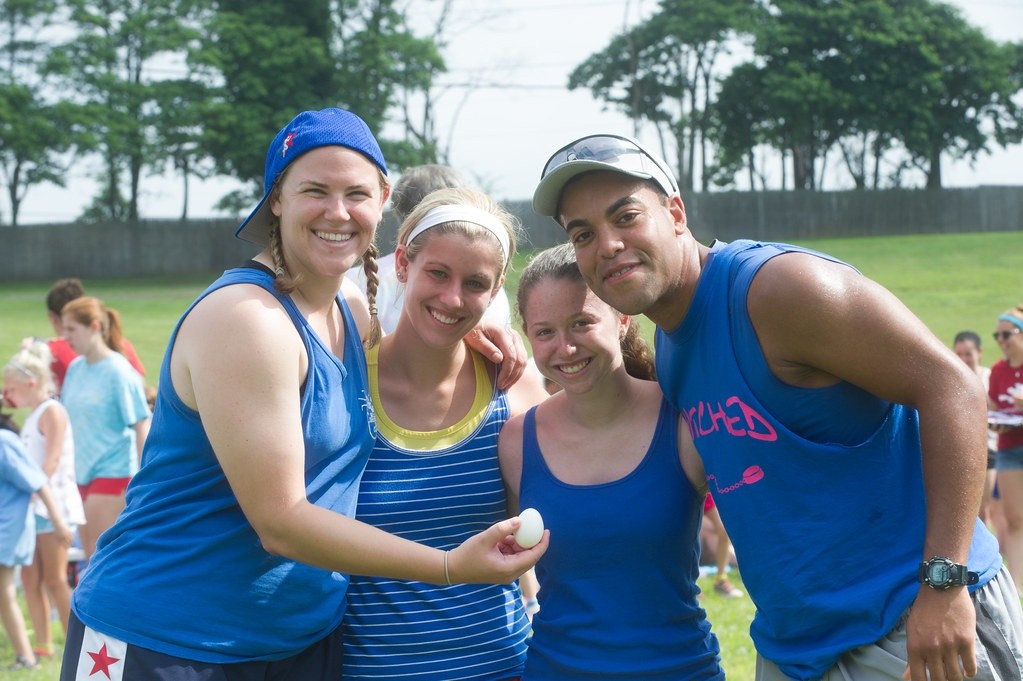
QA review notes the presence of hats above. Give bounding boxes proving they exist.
[234,108,388,249]
[532,132,680,227]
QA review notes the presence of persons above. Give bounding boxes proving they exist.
[3,335,85,660]
[694,487,744,598]
[948,328,1004,551]
[496,239,731,681]
[332,187,545,679]
[983,301,1022,589]
[57,296,152,594]
[54,109,550,679]
[334,164,512,340]
[43,282,147,415]
[0,397,73,669]
[536,127,1021,678]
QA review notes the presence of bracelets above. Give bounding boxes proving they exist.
[442,548,453,586]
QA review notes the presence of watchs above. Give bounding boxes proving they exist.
[916,555,980,592]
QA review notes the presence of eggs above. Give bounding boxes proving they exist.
[513,507,544,549]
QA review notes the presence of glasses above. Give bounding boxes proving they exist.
[992,328,1021,340]
